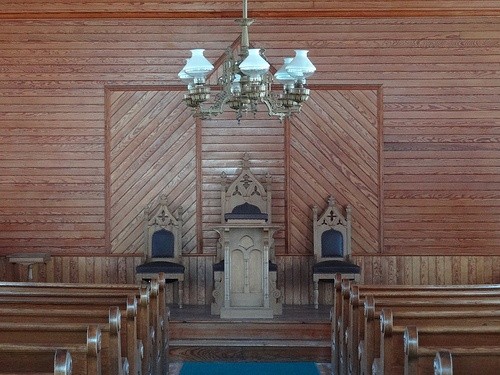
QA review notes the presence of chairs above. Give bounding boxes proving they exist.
[132,193,186,310]
[309,193,362,310]
[210,150,286,318]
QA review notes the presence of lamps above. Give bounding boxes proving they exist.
[175,0,316,126]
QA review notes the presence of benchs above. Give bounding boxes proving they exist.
[0,270,500,375]
